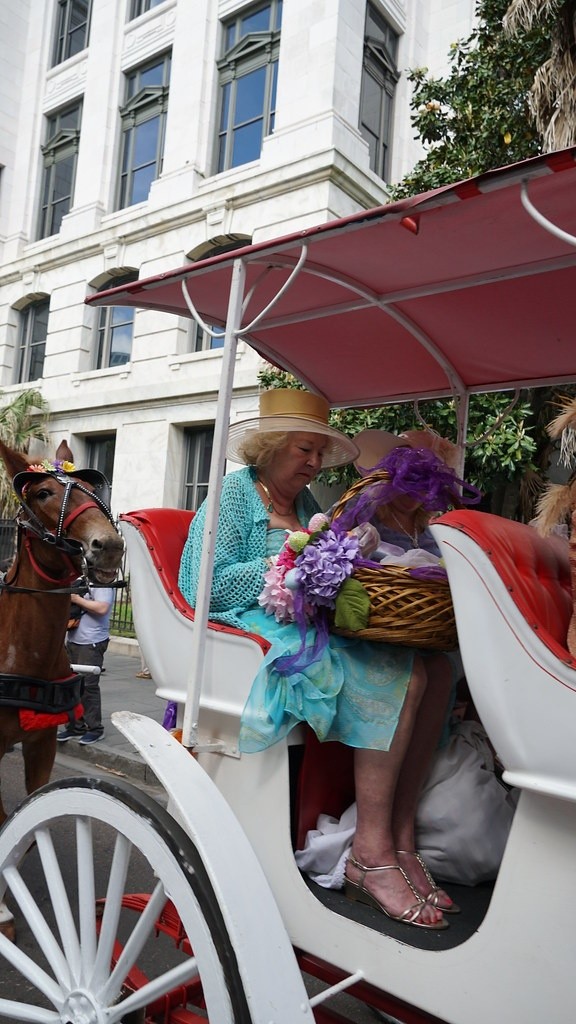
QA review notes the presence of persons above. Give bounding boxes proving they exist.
[136,635,151,680]
[348,428,480,678]
[57,572,115,747]
[179,388,455,930]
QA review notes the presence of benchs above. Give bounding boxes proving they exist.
[114,509,356,855]
[428,510,576,802]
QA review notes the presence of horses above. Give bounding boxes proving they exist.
[0,436,127,944]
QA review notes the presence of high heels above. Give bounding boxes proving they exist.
[396,850,460,912]
[343,851,449,929]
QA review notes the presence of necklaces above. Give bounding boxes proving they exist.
[387,506,418,548]
[257,473,296,518]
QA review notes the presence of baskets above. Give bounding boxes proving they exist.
[313,468,461,648]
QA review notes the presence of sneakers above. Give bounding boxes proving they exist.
[56,730,86,741]
[78,729,106,745]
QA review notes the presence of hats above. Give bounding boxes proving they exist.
[225,389,360,469]
[354,428,481,513]
